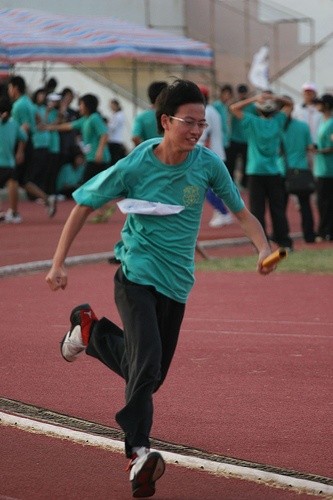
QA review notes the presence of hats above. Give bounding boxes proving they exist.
[199,86,211,96]
[254,99,275,110]
[303,83,317,93]
[47,93,62,101]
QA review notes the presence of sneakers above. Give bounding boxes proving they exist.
[126,447,166,499]
[61,303,97,362]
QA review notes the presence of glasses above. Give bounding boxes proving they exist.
[169,115,209,129]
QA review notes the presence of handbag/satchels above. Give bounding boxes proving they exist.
[284,168,314,195]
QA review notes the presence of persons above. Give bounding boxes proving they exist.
[45,78,277,498]
[0,72,333,255]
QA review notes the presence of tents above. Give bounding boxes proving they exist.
[0,8,217,104]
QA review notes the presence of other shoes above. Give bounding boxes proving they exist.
[90,215,106,223]
[208,211,233,226]
[102,204,117,220]
[0,207,22,223]
[46,194,56,217]
